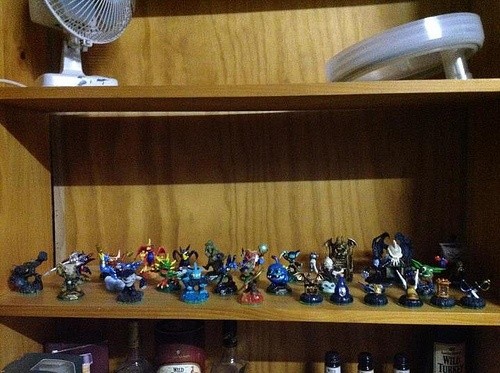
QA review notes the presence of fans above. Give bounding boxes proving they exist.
[34,0,132,86]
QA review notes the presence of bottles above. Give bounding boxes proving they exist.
[210,320,251,373]
[358,352,375,373]
[114,321,153,373]
[393,353,410,373]
[325,352,342,373]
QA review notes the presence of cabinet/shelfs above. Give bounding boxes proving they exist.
[0,0,500,373]
